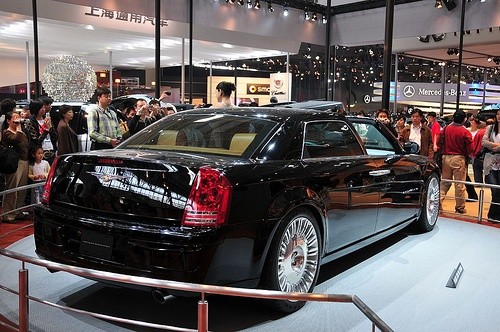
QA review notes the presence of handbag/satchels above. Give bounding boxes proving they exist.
[476,125,493,162]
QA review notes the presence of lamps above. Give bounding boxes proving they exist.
[446,48,459,56]
[224,0,328,26]
[434,0,443,10]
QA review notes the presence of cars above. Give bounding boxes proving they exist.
[37,100,99,156]
[30,104,443,318]
[109,93,178,117]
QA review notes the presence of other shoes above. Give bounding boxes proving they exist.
[456,208,466,213]
[18,216,30,220]
[467,196,478,202]
[2,219,16,224]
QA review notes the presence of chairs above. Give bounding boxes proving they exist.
[228,132,256,151]
[157,129,189,146]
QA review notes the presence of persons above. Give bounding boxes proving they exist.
[330,104,500,224]
[270,97,278,103]
[0,96,58,224]
[194,81,259,109]
[88,87,176,151]
[57,105,79,155]
